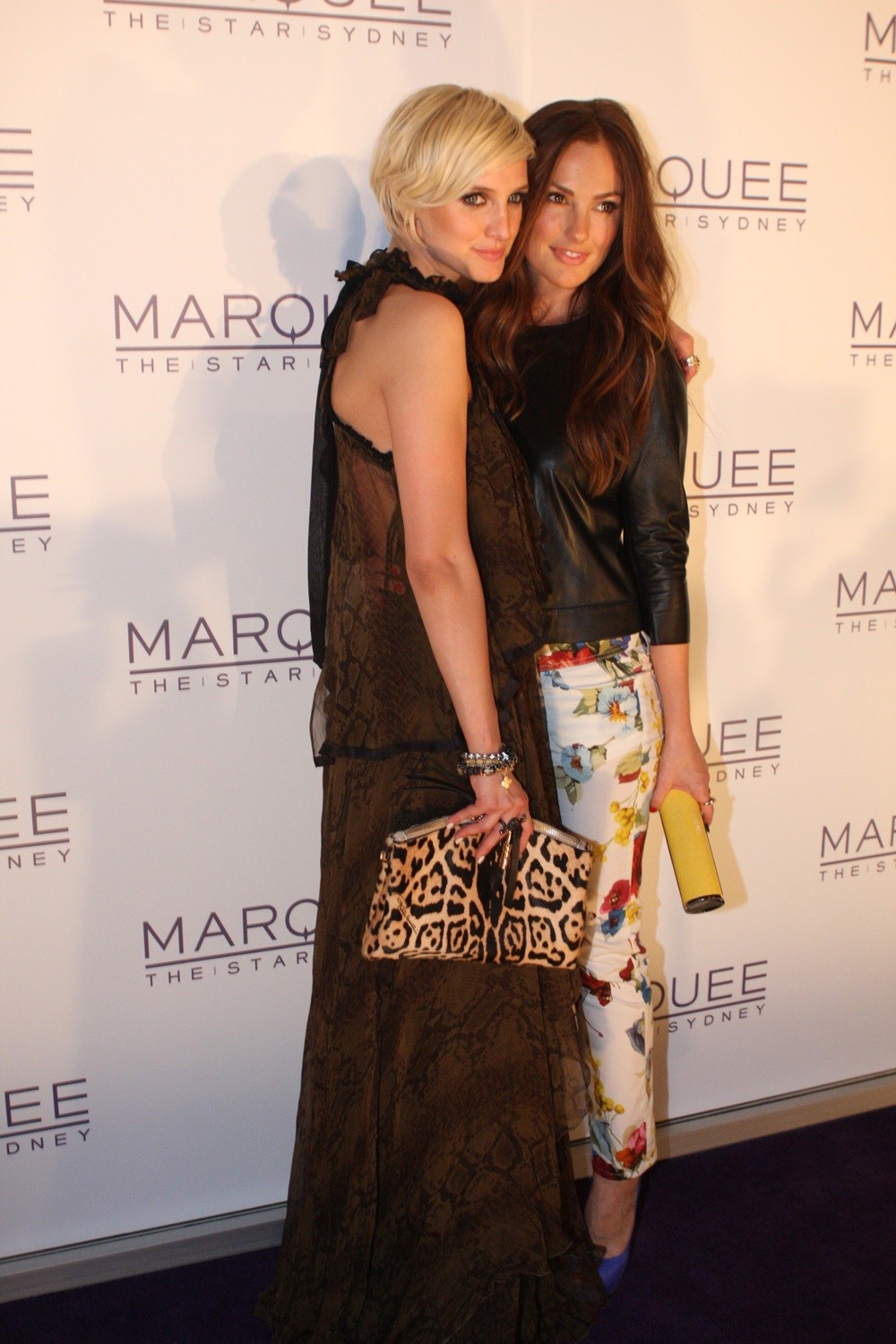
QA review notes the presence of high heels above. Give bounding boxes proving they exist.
[587,1177,637,1291]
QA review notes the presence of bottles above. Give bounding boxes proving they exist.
[659,790,725,913]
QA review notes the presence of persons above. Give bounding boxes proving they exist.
[262,83,700,1344]
[459,95,712,1295]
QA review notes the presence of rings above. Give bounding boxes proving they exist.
[703,797,716,805]
[680,354,700,367]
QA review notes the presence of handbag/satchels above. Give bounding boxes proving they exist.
[359,808,593,972]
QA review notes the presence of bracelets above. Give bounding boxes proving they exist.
[454,743,518,789]
